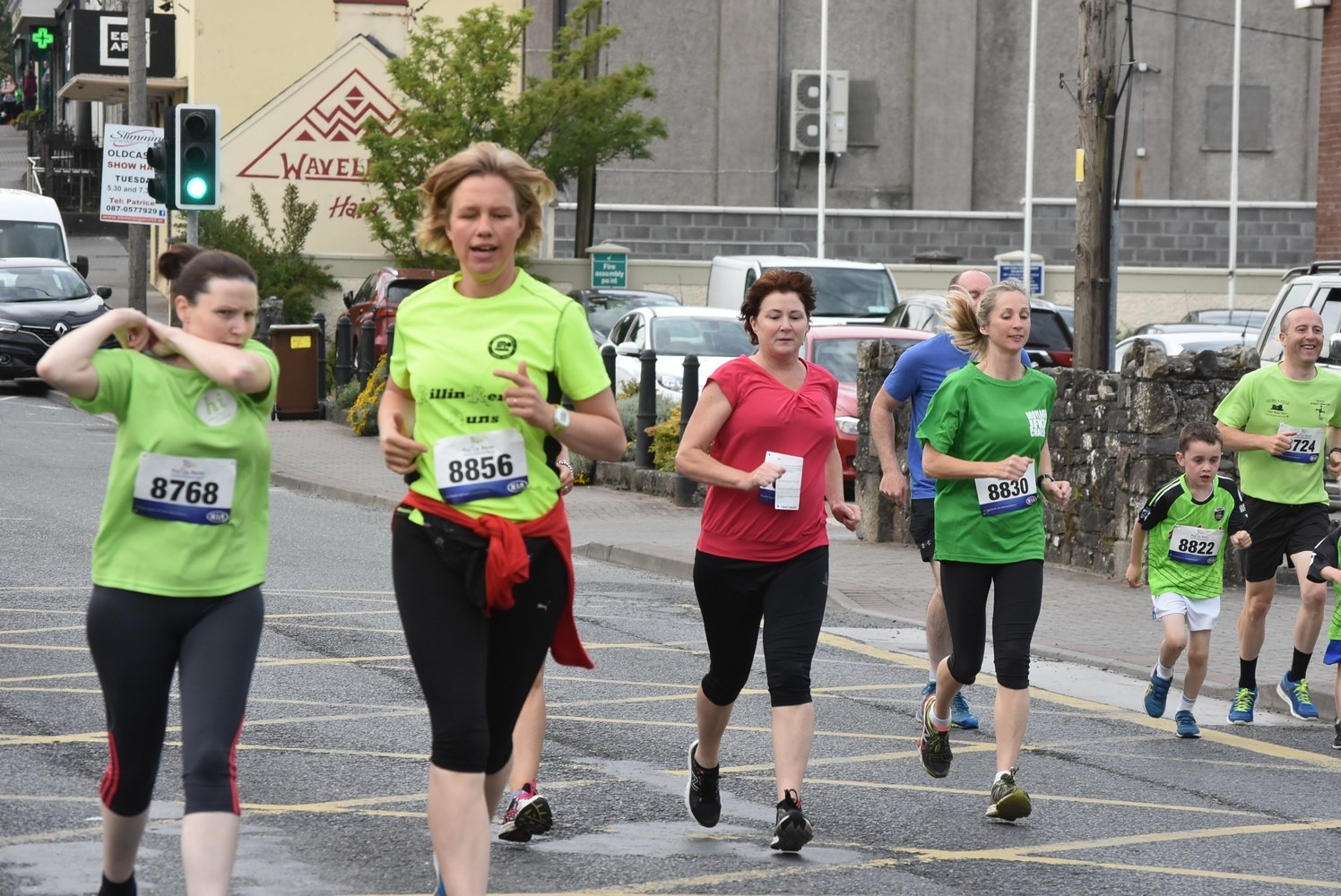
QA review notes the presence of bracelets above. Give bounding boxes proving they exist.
[555,460,574,477]
[1037,474,1055,490]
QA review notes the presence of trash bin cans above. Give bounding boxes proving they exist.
[269,324,325,421]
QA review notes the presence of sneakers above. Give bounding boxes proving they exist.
[950,690,979,729]
[770,789,814,852]
[916,679,937,722]
[498,779,553,843]
[917,695,953,778]
[1142,664,1174,718]
[1227,687,1260,725]
[1332,718,1341,749]
[1276,670,1319,721]
[685,740,726,828]
[986,766,1031,822]
[1174,710,1202,737]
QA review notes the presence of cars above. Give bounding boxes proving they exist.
[566,288,705,352]
[1114,259,1341,372]
[333,268,457,397]
[599,305,760,436]
[798,325,942,483]
[883,295,1073,369]
[0,255,125,382]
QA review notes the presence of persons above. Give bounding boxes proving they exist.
[870,270,1032,727]
[1125,307,1341,749]
[671,268,863,852]
[909,278,1073,820]
[377,141,626,896]
[35,243,279,896]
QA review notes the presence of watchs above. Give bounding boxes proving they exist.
[544,404,571,436]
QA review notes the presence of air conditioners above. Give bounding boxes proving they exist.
[789,70,848,158]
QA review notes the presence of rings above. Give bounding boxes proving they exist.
[564,486,569,492]
[513,398,518,406]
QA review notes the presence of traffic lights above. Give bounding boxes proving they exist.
[174,104,221,210]
[144,107,172,211]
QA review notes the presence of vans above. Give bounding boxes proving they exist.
[1,188,90,280]
[705,255,901,359]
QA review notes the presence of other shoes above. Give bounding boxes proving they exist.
[432,851,447,896]
